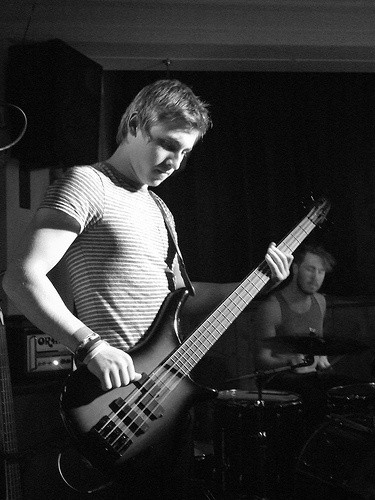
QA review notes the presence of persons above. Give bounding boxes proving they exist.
[3,79,293,500]
[247,247,334,374]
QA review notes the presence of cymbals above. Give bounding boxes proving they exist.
[224,353,315,385]
[259,331,372,358]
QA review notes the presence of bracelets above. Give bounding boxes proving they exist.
[67,327,104,364]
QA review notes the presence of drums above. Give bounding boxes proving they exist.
[327,382,374,424]
[292,415,375,500]
[212,386,304,493]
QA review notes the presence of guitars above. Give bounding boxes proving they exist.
[19,188,335,495]
